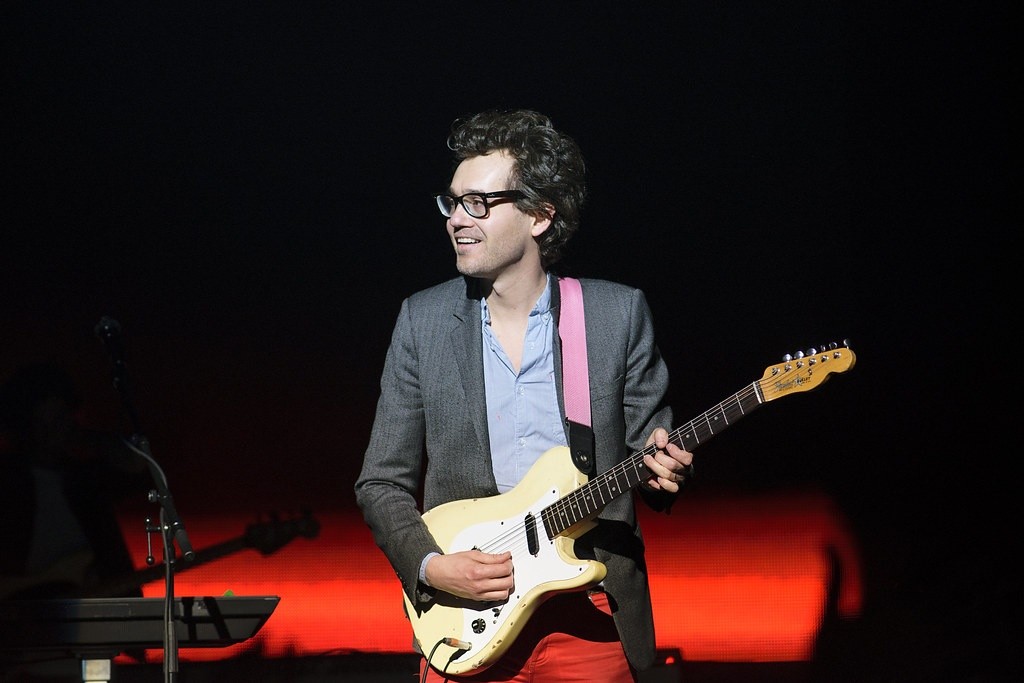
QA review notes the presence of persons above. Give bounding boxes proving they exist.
[354,113,695,683]
[0,363,170,682]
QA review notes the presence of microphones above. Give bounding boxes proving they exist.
[95,314,124,369]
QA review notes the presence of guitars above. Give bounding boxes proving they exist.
[402,337,859,673]
[141,503,325,583]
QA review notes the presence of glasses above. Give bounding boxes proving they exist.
[433,188,526,219]
